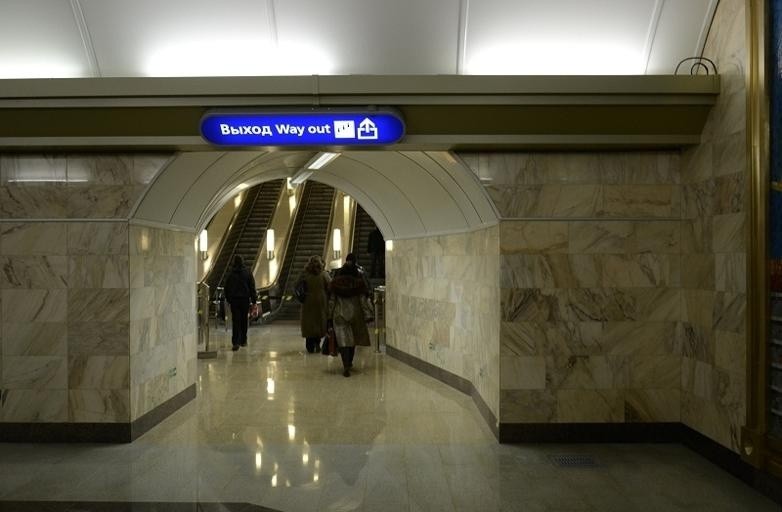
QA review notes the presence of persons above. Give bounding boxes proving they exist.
[225,254,256,350]
[342,253,374,283]
[368,227,385,278]
[296,255,333,351]
[326,263,373,377]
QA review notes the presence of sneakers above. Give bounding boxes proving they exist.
[239,336,248,347]
[307,343,315,353]
[232,343,239,351]
[316,343,322,353]
[343,369,351,377]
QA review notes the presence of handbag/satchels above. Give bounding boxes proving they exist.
[294,272,307,304]
[322,320,340,357]
[249,303,259,322]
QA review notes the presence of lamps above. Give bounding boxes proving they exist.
[265,227,276,262]
[199,228,210,262]
[332,228,342,259]
